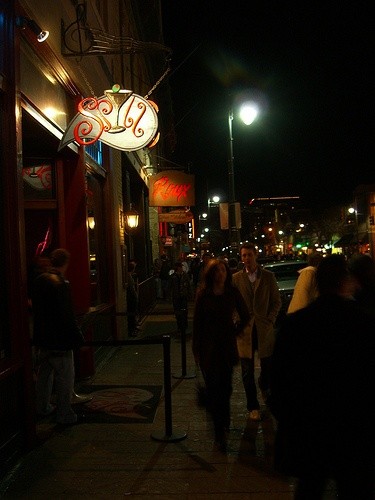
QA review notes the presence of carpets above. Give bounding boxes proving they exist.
[46,384,163,423]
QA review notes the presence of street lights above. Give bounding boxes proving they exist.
[348,207,360,257]
[207,192,222,245]
[225,95,267,255]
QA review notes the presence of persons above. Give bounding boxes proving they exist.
[127,261,142,337]
[153,242,375,500]
[31,248,93,422]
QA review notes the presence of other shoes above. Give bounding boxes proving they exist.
[249,408,262,421]
[71,393,93,405]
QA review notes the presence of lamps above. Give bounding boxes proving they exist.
[15,15,49,42]
[120,209,141,229]
